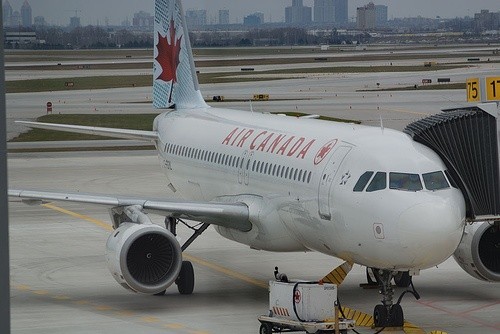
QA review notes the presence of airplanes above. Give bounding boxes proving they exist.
[7,0,499,326]
